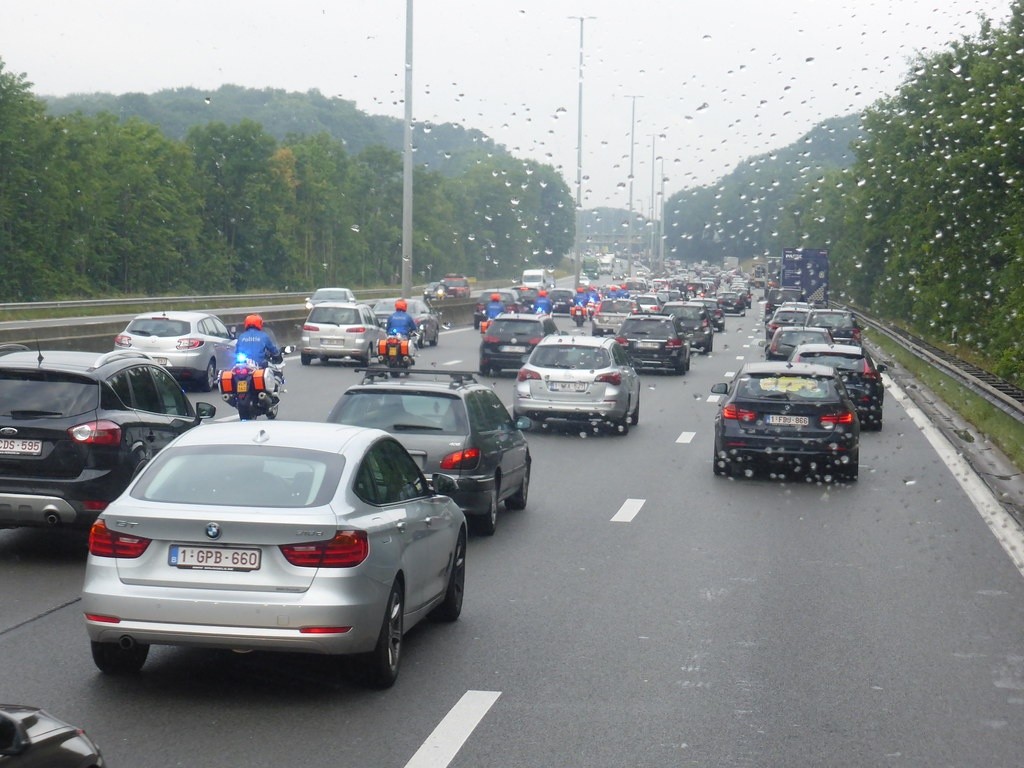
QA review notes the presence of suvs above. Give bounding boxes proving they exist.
[298,301,387,367]
[764,287,806,315]
[800,307,864,346]
[0,341,217,543]
[441,272,470,298]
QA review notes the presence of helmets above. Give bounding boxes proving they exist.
[244,313,264,331]
[611,286,616,290]
[589,286,595,290]
[490,293,500,302]
[577,288,584,293]
[539,291,547,297]
[621,285,626,289]
[394,299,408,312]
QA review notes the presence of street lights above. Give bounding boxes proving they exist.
[567,15,597,289]
[645,132,667,265]
[622,93,645,278]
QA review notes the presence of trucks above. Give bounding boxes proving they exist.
[782,247,829,310]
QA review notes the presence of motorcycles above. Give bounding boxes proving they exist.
[215,343,299,421]
[434,288,447,300]
[376,323,429,375]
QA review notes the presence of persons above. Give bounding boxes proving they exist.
[387,299,417,365]
[486,293,504,320]
[235,313,283,403]
[573,284,630,317]
[534,290,553,316]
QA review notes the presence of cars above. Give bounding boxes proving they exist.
[111,310,238,393]
[777,301,810,310]
[305,287,357,312]
[757,325,836,362]
[323,364,535,539]
[765,306,816,340]
[785,343,887,430]
[423,281,444,300]
[81,418,471,693]
[474,249,753,436]
[709,361,866,480]
[371,298,440,349]
